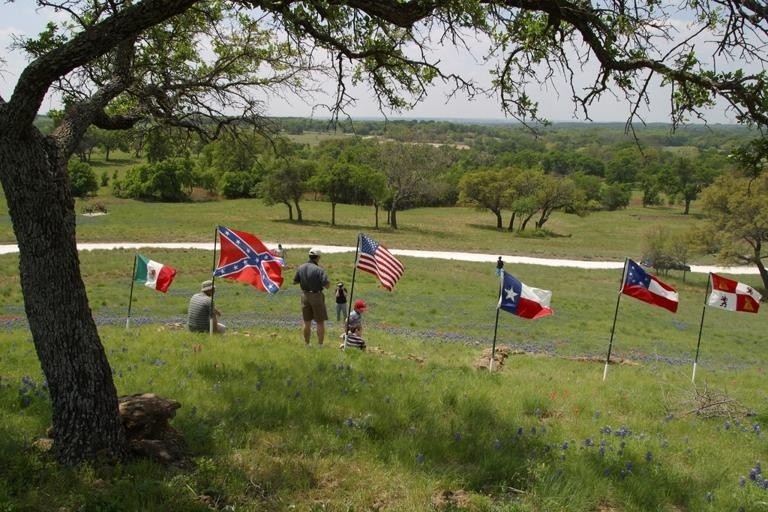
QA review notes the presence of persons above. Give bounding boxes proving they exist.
[277,245,286,258]
[342,324,367,353]
[188,280,221,333]
[496,256,503,278]
[335,282,348,322]
[343,300,369,333]
[292,248,330,345]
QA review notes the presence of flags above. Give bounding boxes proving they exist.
[213,226,284,296]
[620,258,678,314]
[356,233,405,291]
[134,254,176,293]
[708,272,762,313]
[499,269,552,319]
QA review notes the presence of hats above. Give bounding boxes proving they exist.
[353,300,368,312]
[308,248,323,256]
[202,280,216,292]
